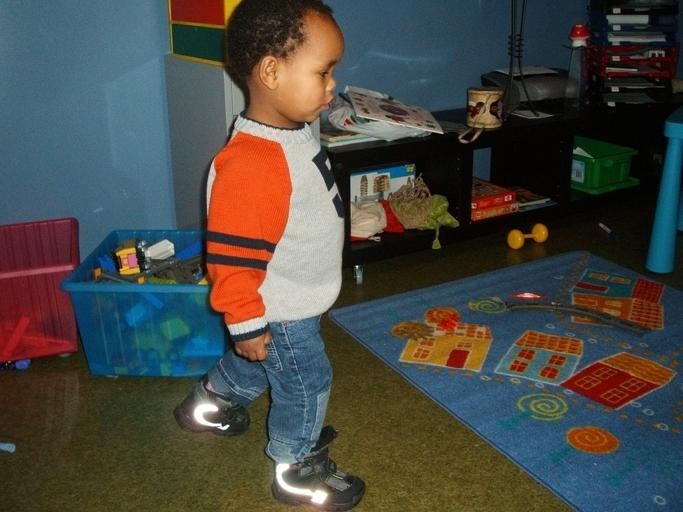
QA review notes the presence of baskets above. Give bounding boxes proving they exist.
[571,135,637,189]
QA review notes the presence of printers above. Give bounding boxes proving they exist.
[481,66,578,123]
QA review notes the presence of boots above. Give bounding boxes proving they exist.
[173,375,249,436]
[271,427,367,510]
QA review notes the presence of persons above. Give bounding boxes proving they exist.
[173,1,367,510]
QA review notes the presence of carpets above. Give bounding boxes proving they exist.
[328,245,682,512]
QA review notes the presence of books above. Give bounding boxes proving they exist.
[320,85,552,222]
[584,16,677,109]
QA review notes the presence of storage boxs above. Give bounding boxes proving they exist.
[567,135,644,198]
[1,216,81,366]
[60,228,230,379]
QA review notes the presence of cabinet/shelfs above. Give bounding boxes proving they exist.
[320,98,682,271]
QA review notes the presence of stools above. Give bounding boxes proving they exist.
[644,103,683,276]
